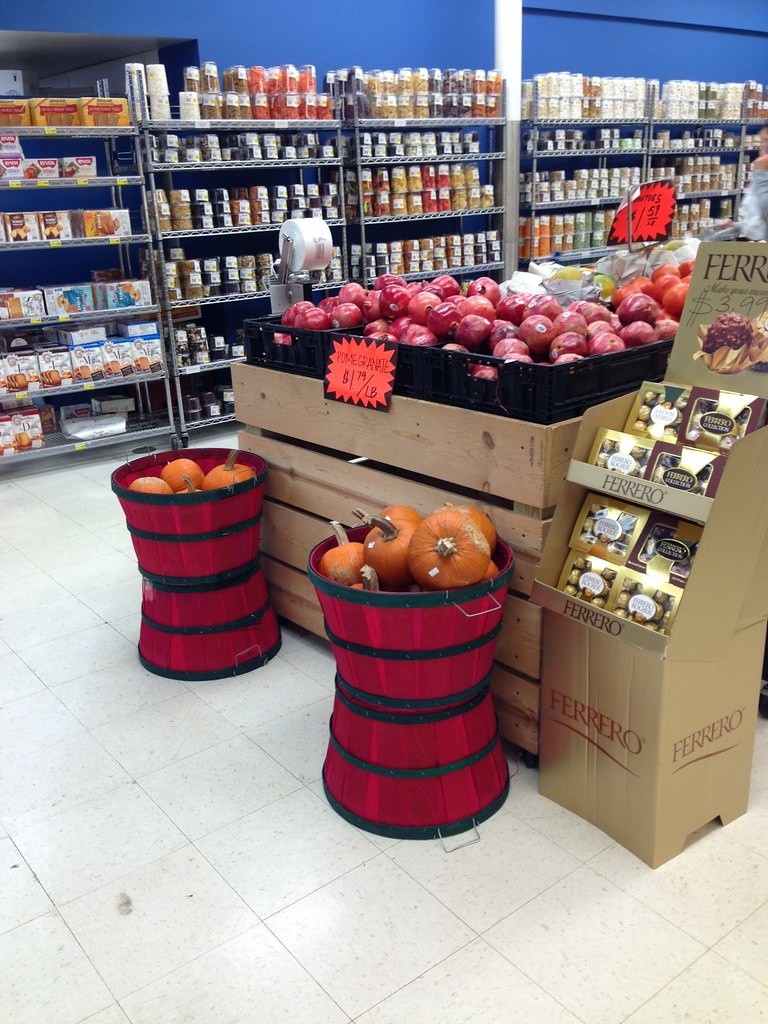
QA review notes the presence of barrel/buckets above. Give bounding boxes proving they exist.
[111,447,282,683]
[307,523,515,853]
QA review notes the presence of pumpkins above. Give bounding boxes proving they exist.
[128,450,254,493]
[319,501,500,593]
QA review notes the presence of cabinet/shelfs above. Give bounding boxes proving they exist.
[0,62,179,459]
[100,78,350,449]
[316,74,507,291]
[487,80,768,279]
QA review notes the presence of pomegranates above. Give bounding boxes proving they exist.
[274,273,680,381]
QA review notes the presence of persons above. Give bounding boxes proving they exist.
[720,127,768,243]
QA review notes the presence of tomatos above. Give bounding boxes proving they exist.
[611,259,697,323]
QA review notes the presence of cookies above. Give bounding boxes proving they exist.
[5,224,150,390]
[15,433,32,446]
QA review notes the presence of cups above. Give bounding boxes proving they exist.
[139,165,754,420]
[520,71,768,120]
[125,63,334,119]
[97,77,110,97]
[142,128,762,163]
[324,67,502,119]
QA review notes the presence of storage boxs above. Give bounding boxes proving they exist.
[526,380,768,869]
[0,69,164,456]
[243,314,677,428]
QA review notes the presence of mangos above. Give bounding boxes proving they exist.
[547,267,617,299]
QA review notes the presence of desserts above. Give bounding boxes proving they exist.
[692,312,768,374]
